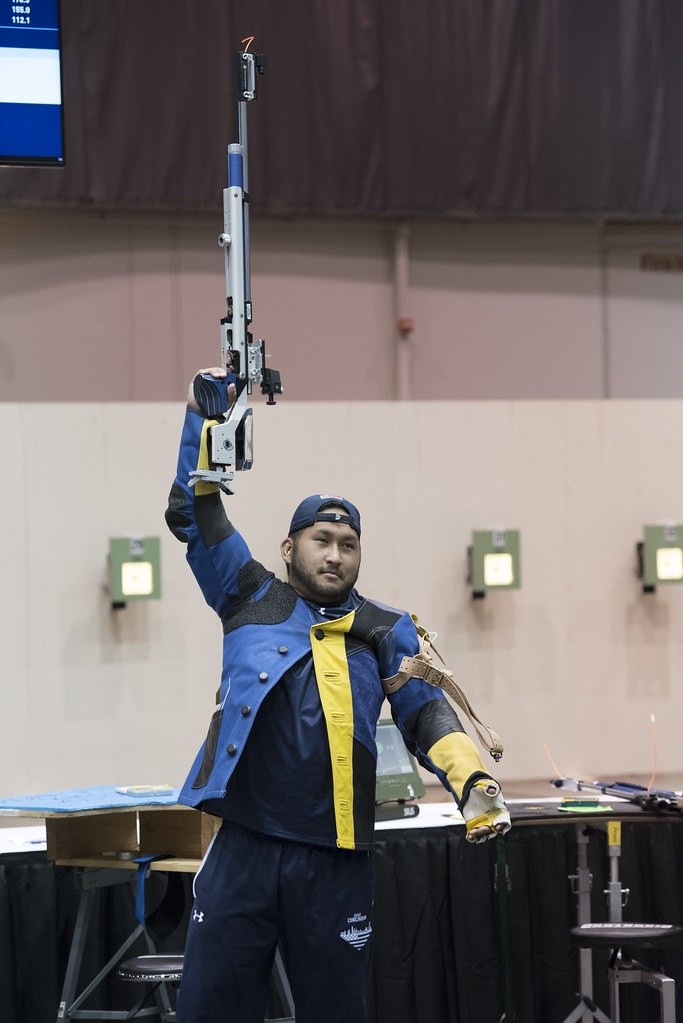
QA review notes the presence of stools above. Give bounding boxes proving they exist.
[116,954,184,1023]
[571,923,683,1023]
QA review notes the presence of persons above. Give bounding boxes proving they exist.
[165,367,511,1023]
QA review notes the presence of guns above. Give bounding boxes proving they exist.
[550,774,683,815]
[186,48,286,496]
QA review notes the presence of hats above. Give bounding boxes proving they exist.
[289,495,361,538]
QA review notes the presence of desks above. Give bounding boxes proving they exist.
[0,774,683,1023]
